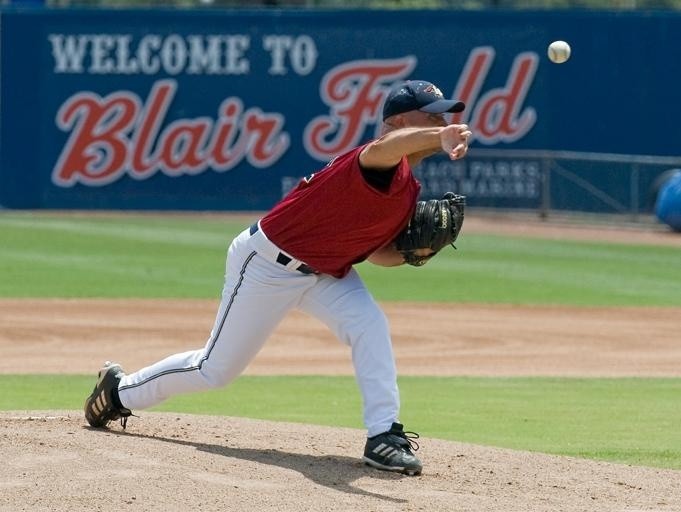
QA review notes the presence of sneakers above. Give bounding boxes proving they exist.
[82,362,139,430]
[362,424,423,477]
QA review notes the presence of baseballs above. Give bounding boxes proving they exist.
[548,40,570,63]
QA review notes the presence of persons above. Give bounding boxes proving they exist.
[81,77,474,477]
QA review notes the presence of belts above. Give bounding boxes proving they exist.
[247,219,317,275]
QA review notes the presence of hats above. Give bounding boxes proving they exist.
[383,79,465,120]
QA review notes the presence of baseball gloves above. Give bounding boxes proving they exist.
[393,192,466,265]
